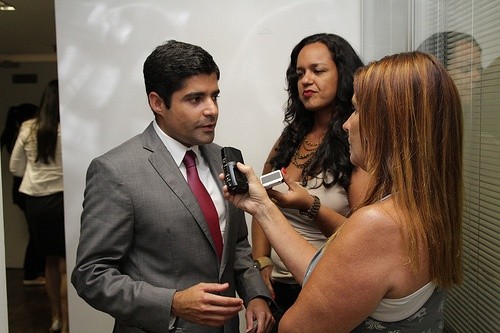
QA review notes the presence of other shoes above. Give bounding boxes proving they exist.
[50,320,61,333]
[23,277,46,285]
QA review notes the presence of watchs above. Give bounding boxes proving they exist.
[252,257,273,269]
[298,196,320,220]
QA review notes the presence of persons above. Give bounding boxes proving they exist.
[418,31,500,333]
[252,34,372,333]
[8,80,70,333]
[72,39,280,333]
[5,103,45,286]
[219,51,465,333]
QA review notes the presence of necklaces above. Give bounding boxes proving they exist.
[293,138,321,168]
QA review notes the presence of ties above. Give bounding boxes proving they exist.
[182,150,224,260]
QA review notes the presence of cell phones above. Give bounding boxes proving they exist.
[259,170,284,189]
